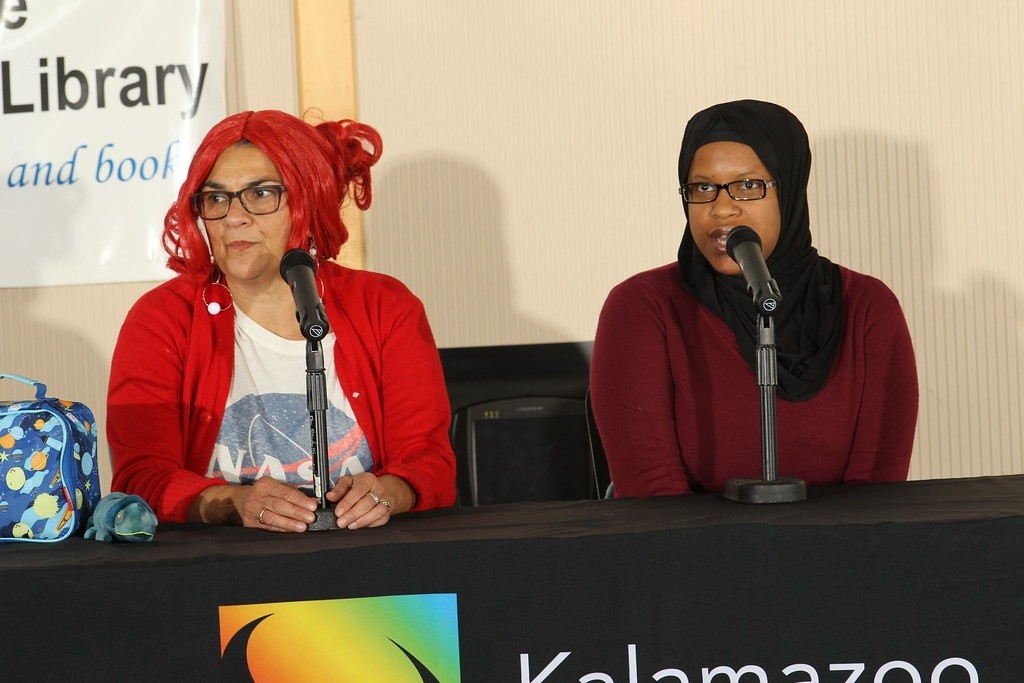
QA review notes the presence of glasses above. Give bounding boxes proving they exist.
[189,185,286,220]
[679,178,776,204]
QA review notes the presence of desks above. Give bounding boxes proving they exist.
[0,473,1024,683]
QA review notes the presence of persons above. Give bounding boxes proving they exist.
[589,99,919,498]
[106,110,458,533]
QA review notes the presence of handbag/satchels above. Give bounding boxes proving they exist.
[0,374,100,543]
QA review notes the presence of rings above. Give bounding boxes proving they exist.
[367,493,380,503]
[259,509,265,524]
[380,500,393,511]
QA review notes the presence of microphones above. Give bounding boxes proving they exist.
[726,226,781,315]
[279,248,329,341]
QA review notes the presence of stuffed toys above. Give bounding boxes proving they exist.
[84,492,158,542]
